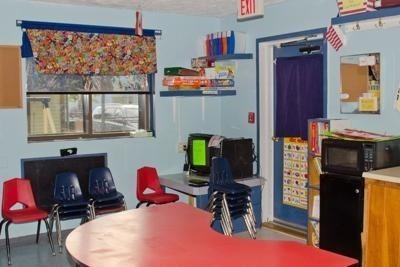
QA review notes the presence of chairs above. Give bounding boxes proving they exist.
[135,165,179,207]
[203,156,258,240]
[0,166,125,266]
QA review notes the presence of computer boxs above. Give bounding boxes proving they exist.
[215,137,253,179]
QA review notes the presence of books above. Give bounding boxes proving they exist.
[307,118,330,248]
[205,30,248,55]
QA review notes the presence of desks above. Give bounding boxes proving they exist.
[65,199,360,266]
[158,173,262,234]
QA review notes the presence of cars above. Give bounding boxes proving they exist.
[93,104,139,132]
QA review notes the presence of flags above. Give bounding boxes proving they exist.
[325,26,343,51]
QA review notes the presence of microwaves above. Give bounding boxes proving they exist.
[319,136,400,175]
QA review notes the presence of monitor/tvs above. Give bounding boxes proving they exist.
[188,133,214,175]
